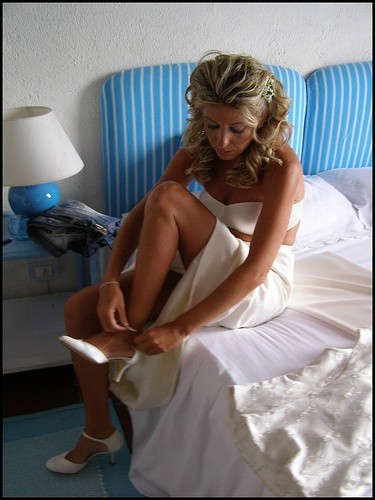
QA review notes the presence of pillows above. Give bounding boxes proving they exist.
[316,167,373,230]
[299,174,371,254]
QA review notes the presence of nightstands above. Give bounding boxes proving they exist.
[2,236,88,376]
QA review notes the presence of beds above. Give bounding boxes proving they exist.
[101,57,373,496]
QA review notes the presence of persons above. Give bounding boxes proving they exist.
[44,47,305,475]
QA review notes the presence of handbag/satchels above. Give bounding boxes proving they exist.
[27,199,121,257]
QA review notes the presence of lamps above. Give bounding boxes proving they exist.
[2,106,85,221]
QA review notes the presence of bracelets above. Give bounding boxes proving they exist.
[99,280,120,293]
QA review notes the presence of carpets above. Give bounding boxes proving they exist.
[2,395,147,498]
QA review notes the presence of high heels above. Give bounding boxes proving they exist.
[45,429,121,475]
[58,334,141,383]
